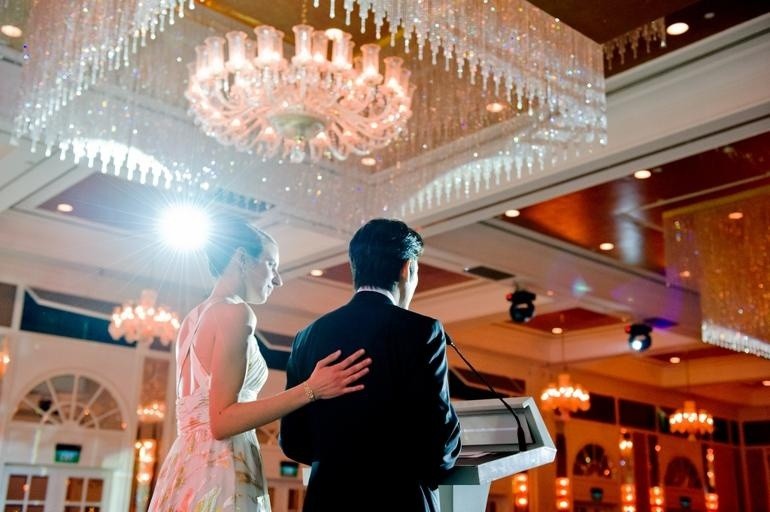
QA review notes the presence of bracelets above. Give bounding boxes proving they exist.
[302,380,316,403]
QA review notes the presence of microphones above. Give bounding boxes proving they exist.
[445,332,527,451]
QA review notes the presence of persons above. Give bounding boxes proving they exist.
[279,217,464,511]
[146,213,372,512]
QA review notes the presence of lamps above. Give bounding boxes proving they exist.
[102,272,181,352]
[11,2,608,233]
[659,188,770,357]
[538,373,719,440]
[501,280,653,357]
